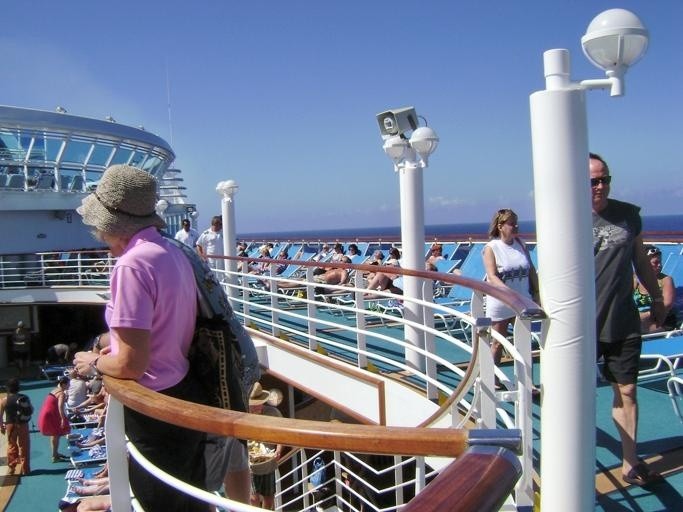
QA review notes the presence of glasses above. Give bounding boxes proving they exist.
[647,248,661,256]
[498,210,505,214]
[591,176,611,186]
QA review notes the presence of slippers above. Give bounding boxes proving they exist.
[623,462,668,491]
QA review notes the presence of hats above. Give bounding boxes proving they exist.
[248,381,270,406]
[76,164,167,236]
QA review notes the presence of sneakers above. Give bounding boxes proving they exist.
[7,467,30,476]
[52,453,65,462]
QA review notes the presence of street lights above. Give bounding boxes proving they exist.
[526,2,651,511]
[374,101,444,381]
[154,195,168,226]
[212,175,250,315]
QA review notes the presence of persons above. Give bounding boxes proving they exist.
[2,320,110,511]
[72,163,251,511]
[235,240,450,298]
[308,409,372,511]
[589,154,677,491]
[483,209,540,391]
[248,383,284,512]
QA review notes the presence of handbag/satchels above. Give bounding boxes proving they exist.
[189,314,247,447]
[529,266,541,307]
[16,395,31,422]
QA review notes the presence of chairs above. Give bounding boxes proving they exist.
[41,359,110,512]
[0,173,83,191]
